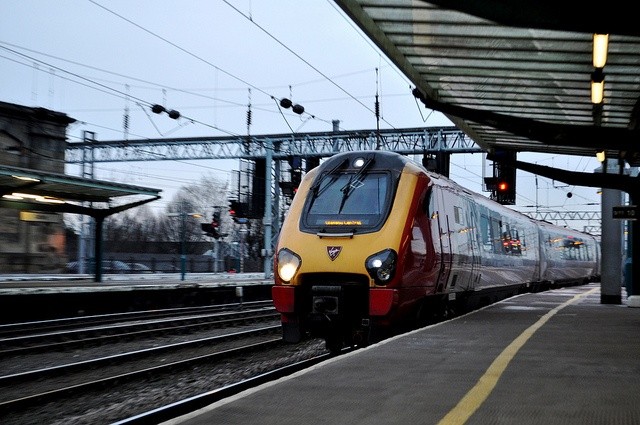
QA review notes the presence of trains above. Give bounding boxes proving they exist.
[272,151,601,355]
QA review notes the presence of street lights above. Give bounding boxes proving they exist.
[168,213,203,281]
[232,218,251,259]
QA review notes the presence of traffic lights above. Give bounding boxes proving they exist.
[291,170,302,200]
[437,150,449,177]
[228,200,236,214]
[213,212,220,226]
[484,146,516,205]
